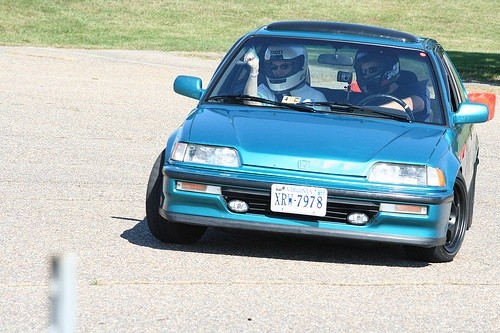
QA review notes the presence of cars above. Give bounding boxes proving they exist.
[145,20,491,264]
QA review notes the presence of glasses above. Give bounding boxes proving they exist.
[361,66,378,75]
[270,64,291,70]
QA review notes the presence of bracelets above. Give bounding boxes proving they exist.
[249,72,259,77]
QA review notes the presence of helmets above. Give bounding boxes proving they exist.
[354,48,400,96]
[264,43,308,95]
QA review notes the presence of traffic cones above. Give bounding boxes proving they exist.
[349,81,361,92]
[467,92,496,123]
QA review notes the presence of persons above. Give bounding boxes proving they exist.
[243,43,331,112]
[353,47,430,122]
